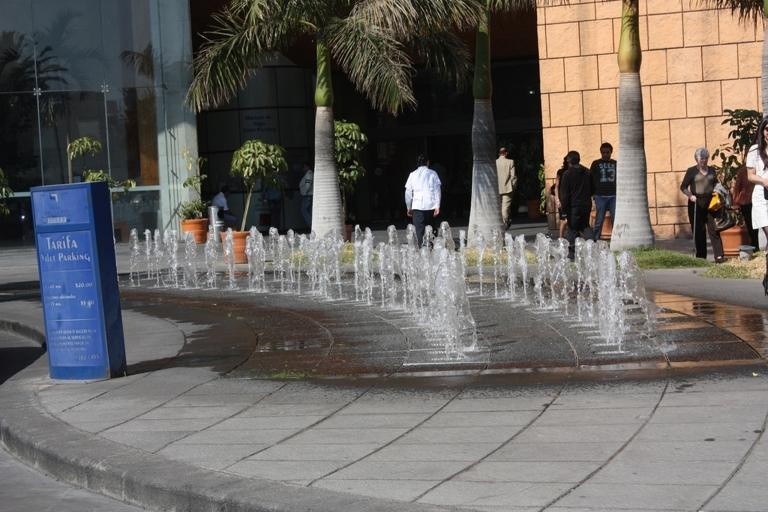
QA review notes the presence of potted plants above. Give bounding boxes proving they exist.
[168,143,212,245]
[709,105,767,258]
[215,140,285,265]
[330,118,366,240]
[522,148,548,220]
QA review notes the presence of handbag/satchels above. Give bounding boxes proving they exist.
[707,195,735,232]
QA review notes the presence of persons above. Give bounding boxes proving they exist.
[554,156,571,238]
[211,185,239,224]
[495,147,517,230]
[262,169,288,225]
[558,151,595,260]
[680,147,728,264]
[299,162,314,227]
[733,166,760,253]
[404,153,442,250]
[745,115,768,294]
[590,143,617,242]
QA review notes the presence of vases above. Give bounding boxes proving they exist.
[589,210,614,240]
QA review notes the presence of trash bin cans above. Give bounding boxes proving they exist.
[207,205,225,252]
